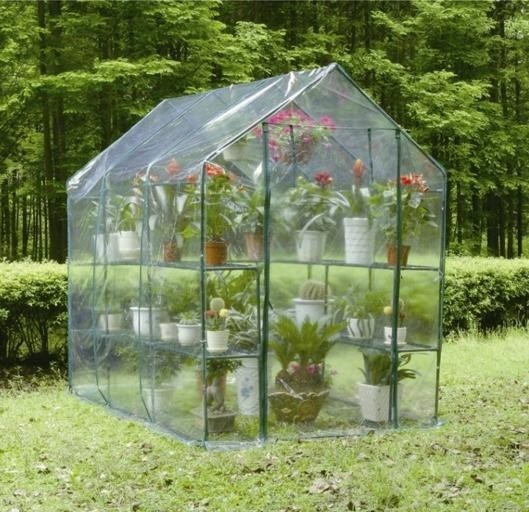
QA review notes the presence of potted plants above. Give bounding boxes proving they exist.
[229,177,273,261]
[324,283,390,341]
[321,159,379,266]
[382,297,408,348]
[182,162,239,266]
[194,354,238,432]
[114,177,136,259]
[159,315,178,342]
[135,159,185,264]
[81,200,114,261]
[368,172,437,266]
[285,177,333,263]
[177,311,202,348]
[356,348,423,423]
[129,282,161,339]
[268,309,352,426]
[227,305,263,416]
[291,280,335,334]
[205,297,231,353]
[122,340,189,417]
